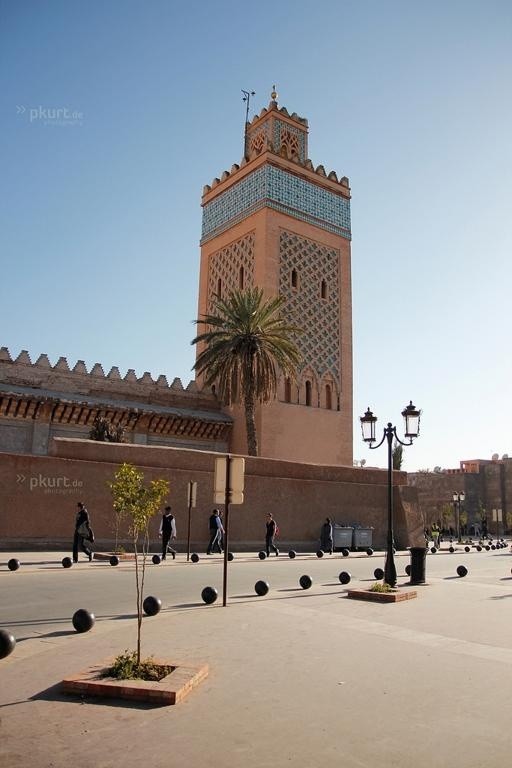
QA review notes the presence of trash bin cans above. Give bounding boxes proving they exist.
[333,527,375,552]
[406,546,429,584]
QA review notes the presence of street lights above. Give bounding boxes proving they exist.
[450,490,465,542]
[360,401,421,587]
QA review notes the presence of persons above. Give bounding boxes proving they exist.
[72,502,95,563]
[430,522,440,549]
[265,512,280,557]
[442,513,489,540]
[424,528,429,547]
[206,508,226,555]
[158,506,176,560]
[321,517,334,555]
